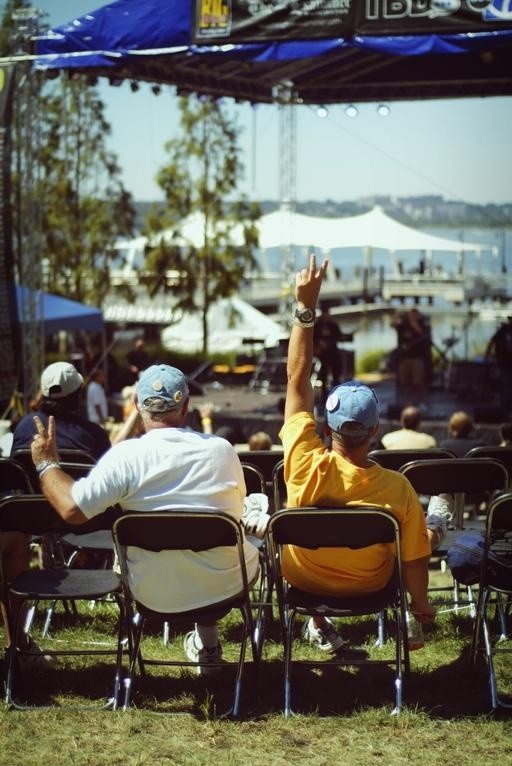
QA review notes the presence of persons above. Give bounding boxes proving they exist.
[279,253,454,653]
[31,365,271,678]
[198,402,271,450]
[11,362,122,569]
[311,301,512,460]
[87,340,148,424]
[0,531,51,672]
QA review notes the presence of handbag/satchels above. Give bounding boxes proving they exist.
[446,529,512,585]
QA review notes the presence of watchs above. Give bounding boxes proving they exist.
[35,459,58,476]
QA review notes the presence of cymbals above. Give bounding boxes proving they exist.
[442,338,459,343]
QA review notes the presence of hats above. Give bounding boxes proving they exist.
[39,361,83,399]
[135,364,190,413]
[326,382,379,437]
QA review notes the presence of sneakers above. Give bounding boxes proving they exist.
[239,492,271,539]
[426,493,458,554]
[4,634,43,673]
[301,615,345,653]
[183,630,224,674]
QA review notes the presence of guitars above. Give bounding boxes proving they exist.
[312,333,352,356]
[386,328,430,370]
